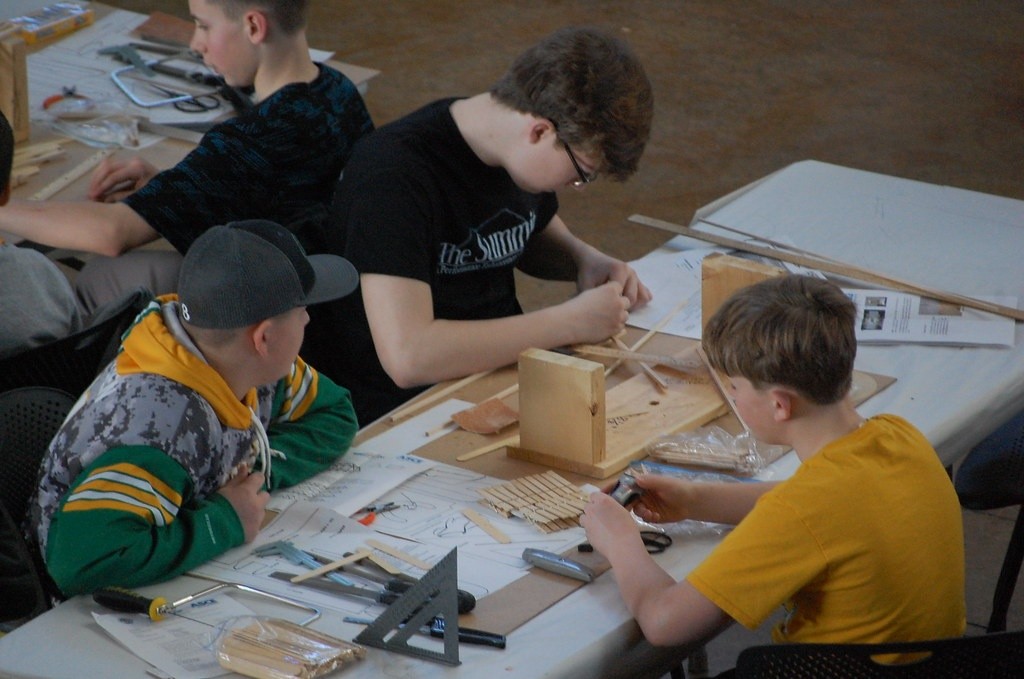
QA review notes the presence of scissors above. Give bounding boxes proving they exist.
[148,82,220,113]
[639,529,673,555]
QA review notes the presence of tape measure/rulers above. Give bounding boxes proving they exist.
[352,543,463,667]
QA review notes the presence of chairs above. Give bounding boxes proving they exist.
[734,631,1024,677]
[953,414,1023,632]
[0,294,139,401]
[0,388,79,636]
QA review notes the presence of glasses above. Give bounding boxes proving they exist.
[548,117,598,192]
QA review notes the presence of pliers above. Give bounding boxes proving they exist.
[351,501,401,526]
[41,85,95,110]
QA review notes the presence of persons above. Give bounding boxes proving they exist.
[308,24,654,436]
[0,0,378,362]
[576,275,966,665]
[20,218,359,610]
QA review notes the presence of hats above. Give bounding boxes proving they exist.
[176,218,360,330]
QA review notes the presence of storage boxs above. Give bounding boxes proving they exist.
[4,2,93,43]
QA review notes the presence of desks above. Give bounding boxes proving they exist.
[1,159,1024,679]
[0,1,369,281]
